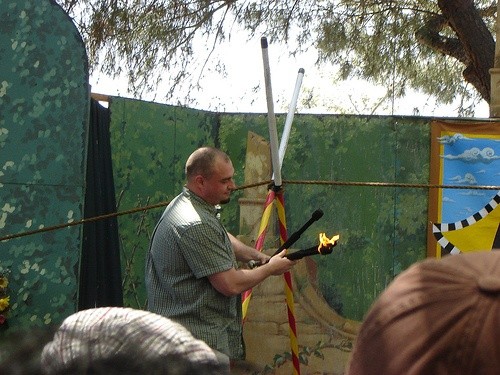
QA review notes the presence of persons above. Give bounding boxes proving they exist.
[144,146,300,366]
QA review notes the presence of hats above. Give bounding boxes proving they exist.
[40,307,230,375]
[346,249,500,375]
